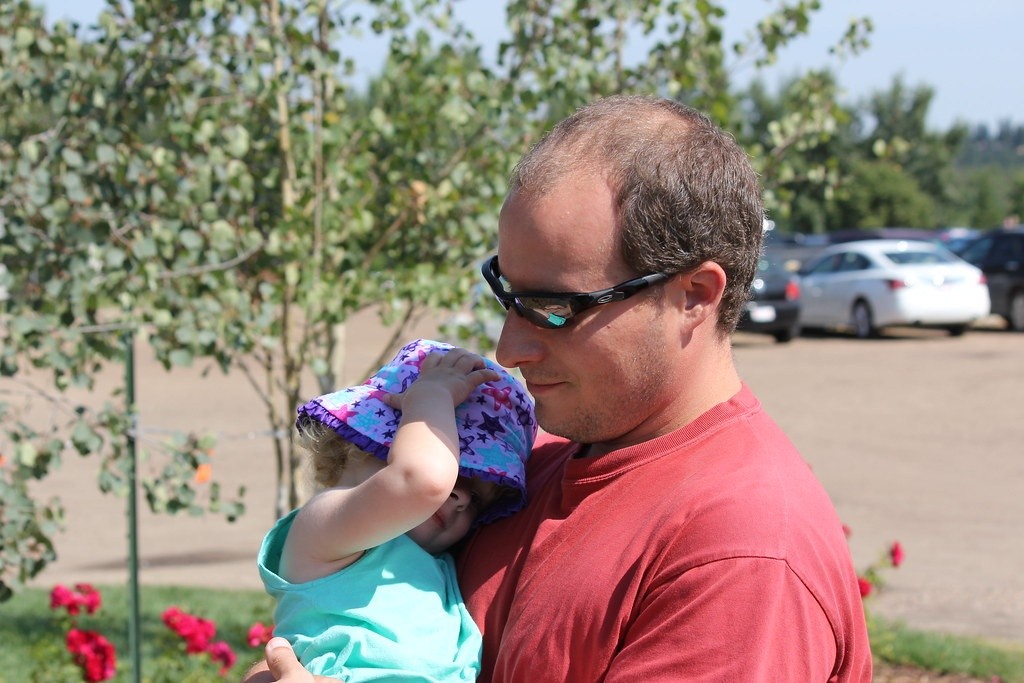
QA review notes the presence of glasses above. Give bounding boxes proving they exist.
[481,254,702,329]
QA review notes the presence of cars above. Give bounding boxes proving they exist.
[798,237,992,340]
[957,227,1024,333]
[759,229,949,266]
[734,259,800,342]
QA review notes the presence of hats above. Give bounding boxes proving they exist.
[296,339,537,520]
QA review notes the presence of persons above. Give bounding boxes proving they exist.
[257,338,539,683]
[240,93,874,683]
